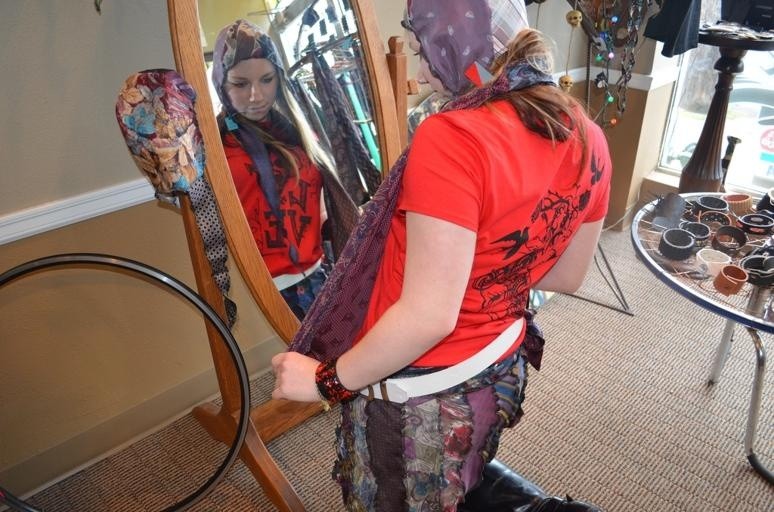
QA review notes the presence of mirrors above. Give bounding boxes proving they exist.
[167,0,422,510]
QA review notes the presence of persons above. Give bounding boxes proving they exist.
[215,13,339,332]
[270,0,612,511]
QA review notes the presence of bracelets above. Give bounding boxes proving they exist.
[312,355,358,403]
[656,177,773,296]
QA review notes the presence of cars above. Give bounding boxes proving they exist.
[657,80,773,188]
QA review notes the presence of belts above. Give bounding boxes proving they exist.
[359,320,524,404]
[272,260,322,291]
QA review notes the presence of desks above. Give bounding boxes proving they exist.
[629,190,774,491]
